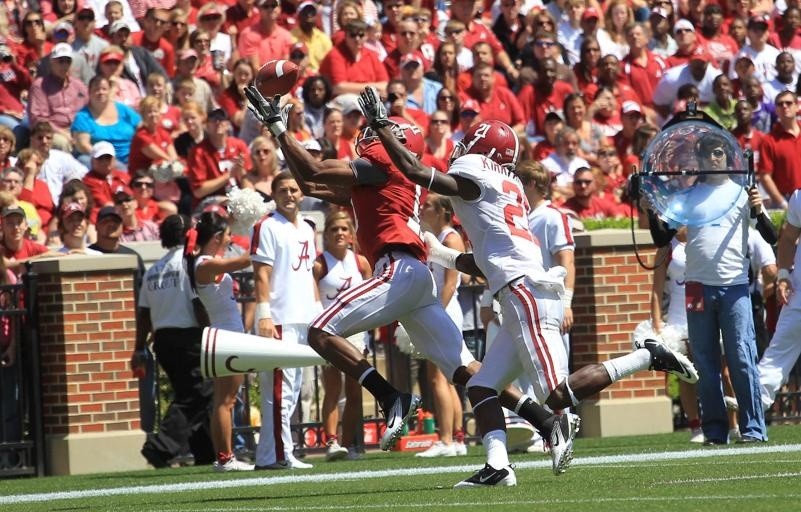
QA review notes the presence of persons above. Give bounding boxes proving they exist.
[243,86,582,476]
[356,85,700,487]
[0,0,454,352]
[618,137,801,445]
[515,156,582,452]
[135,173,468,469]
[425,1,799,216]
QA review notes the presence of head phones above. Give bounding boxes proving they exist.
[694,132,735,167]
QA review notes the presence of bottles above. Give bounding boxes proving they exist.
[417,408,435,435]
[401,422,408,435]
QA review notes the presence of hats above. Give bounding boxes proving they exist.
[691,43,712,63]
[748,16,771,28]
[399,53,422,69]
[92,141,115,159]
[459,98,480,113]
[51,6,130,63]
[289,0,317,55]
[2,205,24,219]
[581,8,599,20]
[621,101,642,114]
[62,185,133,220]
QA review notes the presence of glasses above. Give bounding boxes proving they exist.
[116,197,132,204]
[350,32,365,37]
[134,182,154,187]
[702,150,723,159]
[432,97,477,123]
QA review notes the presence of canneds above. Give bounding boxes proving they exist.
[402,424,408,437]
[423,418,435,433]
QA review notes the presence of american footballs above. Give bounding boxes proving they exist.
[255,60,299,97]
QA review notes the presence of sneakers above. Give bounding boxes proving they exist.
[454,463,516,487]
[690,427,758,445]
[326,439,348,460]
[415,441,467,458]
[635,336,700,385]
[212,453,313,472]
[545,413,581,476]
[381,392,424,451]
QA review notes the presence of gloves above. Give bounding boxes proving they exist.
[244,86,293,138]
[358,86,388,130]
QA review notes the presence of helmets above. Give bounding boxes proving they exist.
[448,119,520,171]
[355,116,423,165]
